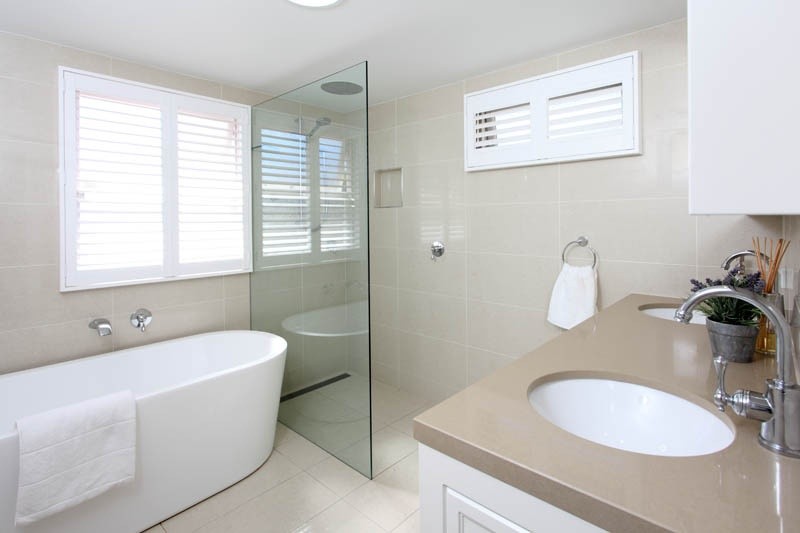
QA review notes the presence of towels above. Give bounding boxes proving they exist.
[546,263,599,330]
[14,387,139,528]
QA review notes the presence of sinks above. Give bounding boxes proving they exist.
[638,303,708,325]
[526,369,737,458]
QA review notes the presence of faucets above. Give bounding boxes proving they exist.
[675,285,800,460]
[720,248,787,332]
[89,318,113,337]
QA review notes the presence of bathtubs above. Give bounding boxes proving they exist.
[2,328,292,533]
[281,299,370,423]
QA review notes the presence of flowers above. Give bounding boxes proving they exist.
[690,267,767,330]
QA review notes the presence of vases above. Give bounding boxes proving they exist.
[705,317,760,364]
